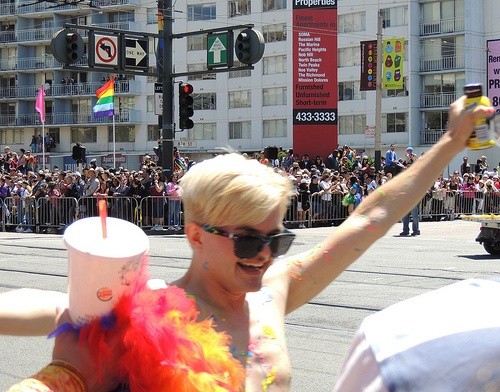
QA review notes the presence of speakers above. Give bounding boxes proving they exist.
[72,146,86,161]
[264,147,278,159]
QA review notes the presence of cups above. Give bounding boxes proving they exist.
[63,217,148,330]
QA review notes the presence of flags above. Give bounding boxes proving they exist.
[93,79,114,119]
[36,86,45,123]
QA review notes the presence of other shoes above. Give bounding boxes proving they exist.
[412,231,420,235]
[23,228,33,232]
[175,226,181,231]
[400,232,409,236]
[151,226,164,231]
[168,226,176,230]
[299,224,306,228]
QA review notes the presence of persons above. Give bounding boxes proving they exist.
[61,77,77,85]
[30,133,54,153]
[243,144,417,229]
[420,156,500,221]
[0,147,196,235]
[102,74,128,90]
[0,95,495,392]
[400,204,420,236]
[5,305,129,392]
[330,277,500,392]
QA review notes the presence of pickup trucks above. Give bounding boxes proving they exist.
[474,218,500,257]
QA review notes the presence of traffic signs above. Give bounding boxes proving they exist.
[124,35,150,70]
[206,30,235,68]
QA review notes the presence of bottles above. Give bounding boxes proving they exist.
[463,83,495,151]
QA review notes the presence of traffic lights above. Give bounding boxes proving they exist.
[178,82,195,130]
[234,28,265,65]
[50,27,86,65]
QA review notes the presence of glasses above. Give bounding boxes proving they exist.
[196,222,296,259]
